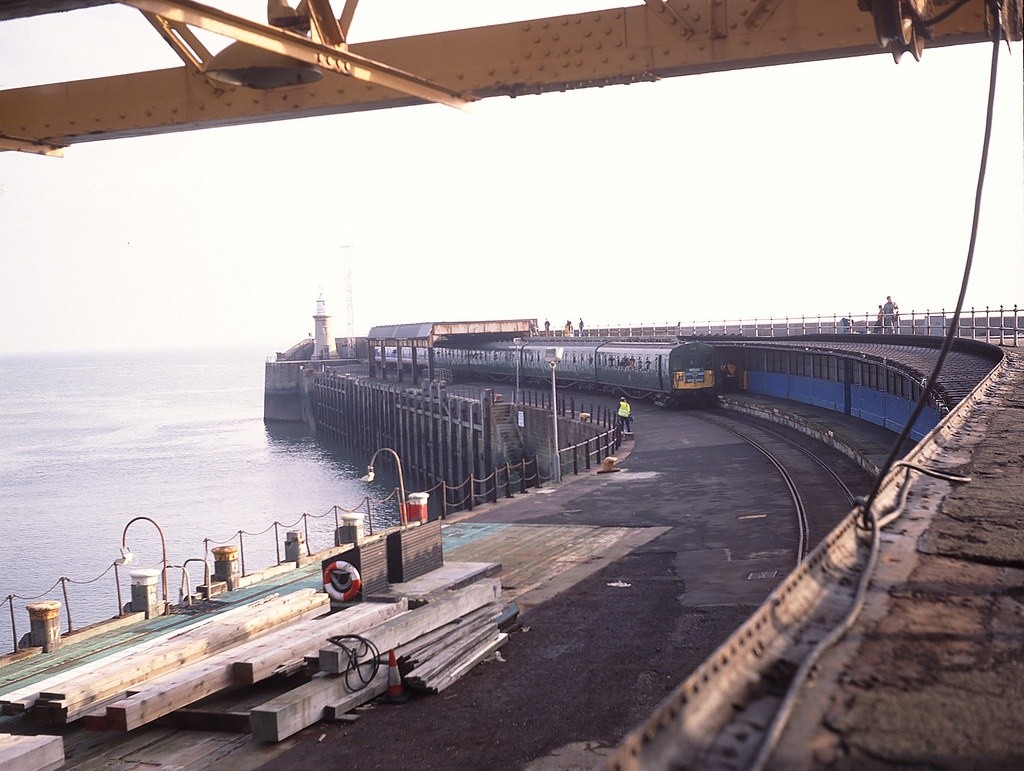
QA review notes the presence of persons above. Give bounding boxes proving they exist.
[617,396,631,437]
[528,317,584,337]
[873,295,899,333]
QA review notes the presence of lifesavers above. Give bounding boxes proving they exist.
[323,561,361,601]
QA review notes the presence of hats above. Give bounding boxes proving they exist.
[621,397,625,399]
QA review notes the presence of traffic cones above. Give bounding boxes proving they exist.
[385,650,410,703]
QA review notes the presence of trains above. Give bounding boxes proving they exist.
[373,341,721,409]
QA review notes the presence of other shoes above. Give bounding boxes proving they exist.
[891,330,895,334]
